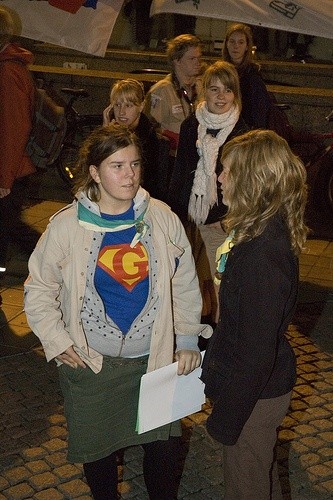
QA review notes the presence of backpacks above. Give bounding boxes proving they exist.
[2,58,67,169]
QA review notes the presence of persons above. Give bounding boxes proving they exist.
[198,129,308,500]
[0,9,268,500]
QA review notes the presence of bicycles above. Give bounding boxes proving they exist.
[58,88,104,190]
[272,102,333,211]
